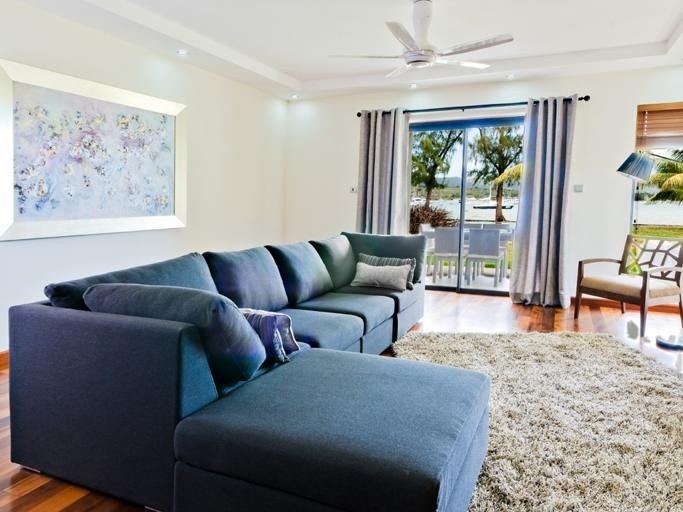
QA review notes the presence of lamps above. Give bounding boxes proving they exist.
[616,151,683,185]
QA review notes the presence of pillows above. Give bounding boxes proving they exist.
[234,306,300,365]
[346,251,415,291]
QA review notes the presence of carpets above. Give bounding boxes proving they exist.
[395,329,682,510]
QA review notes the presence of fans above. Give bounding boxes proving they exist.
[325,0,516,78]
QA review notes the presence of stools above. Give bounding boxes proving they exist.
[170,345,493,512]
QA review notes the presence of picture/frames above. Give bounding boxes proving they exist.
[1,59,189,241]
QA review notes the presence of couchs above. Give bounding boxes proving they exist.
[5,231,428,512]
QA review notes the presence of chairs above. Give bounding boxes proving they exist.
[574,235,682,336]
[421,223,515,289]
